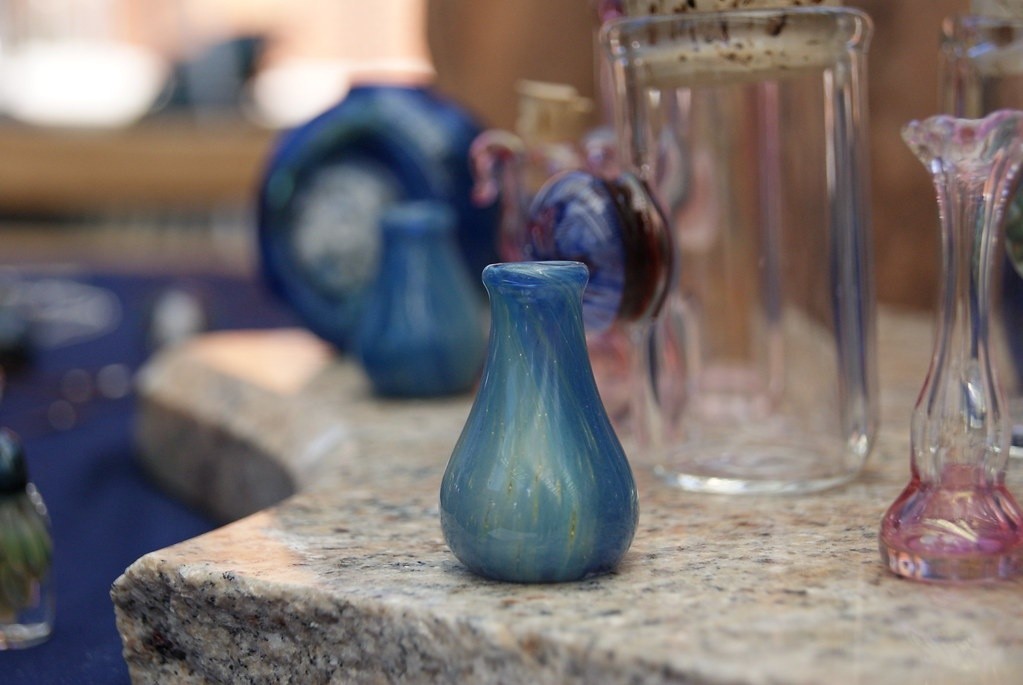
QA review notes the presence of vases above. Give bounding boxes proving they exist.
[582,7,877,500]
[879,109,1023,587]
[440,262,641,585]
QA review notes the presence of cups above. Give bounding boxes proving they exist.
[594,7,878,496]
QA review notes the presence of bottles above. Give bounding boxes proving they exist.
[438,260,639,585]
[878,109,1023,582]
[360,204,486,398]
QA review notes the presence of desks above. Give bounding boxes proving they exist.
[109,308,1023,685]
